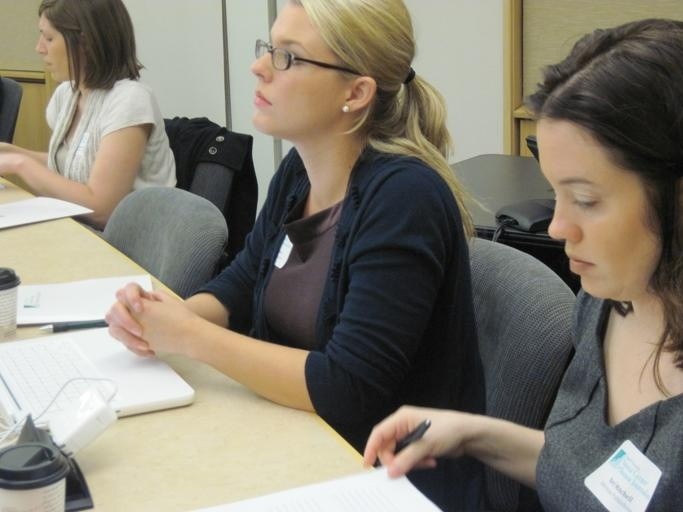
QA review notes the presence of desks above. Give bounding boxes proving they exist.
[0,169,447,512]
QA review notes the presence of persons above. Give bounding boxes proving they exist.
[102,0,490,512]
[0,0,180,241]
[361,17,681,512]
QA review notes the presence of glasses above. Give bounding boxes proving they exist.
[254,38,360,78]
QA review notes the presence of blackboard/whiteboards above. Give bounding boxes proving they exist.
[123,0,522,222]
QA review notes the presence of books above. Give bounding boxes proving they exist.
[0,326,196,426]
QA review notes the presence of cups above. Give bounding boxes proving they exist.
[1,267,21,344]
[0,446,71,512]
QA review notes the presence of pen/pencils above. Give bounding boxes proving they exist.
[38,320,109,332]
[373,419,431,467]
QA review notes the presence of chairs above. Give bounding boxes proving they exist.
[465,234,578,510]
[164,118,257,271]
[100,187,230,301]
[0,77,25,145]
